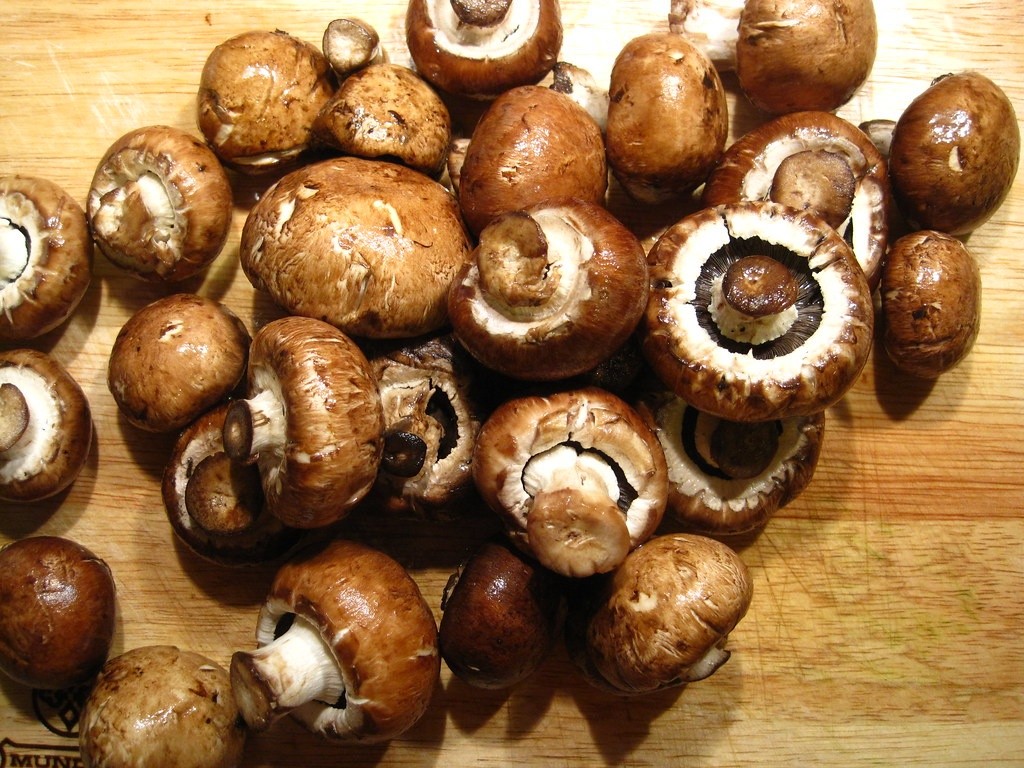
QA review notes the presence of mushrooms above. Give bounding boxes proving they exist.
[0,0,1019,767]
